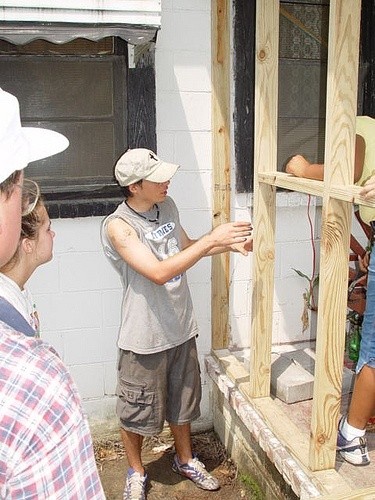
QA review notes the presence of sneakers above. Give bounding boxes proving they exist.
[122,467,148,500]
[336,413,371,465]
[170,454,220,491]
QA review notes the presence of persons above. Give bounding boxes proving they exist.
[0,88,108,499]
[0,190,56,338]
[100,148,253,499]
[285,115,375,468]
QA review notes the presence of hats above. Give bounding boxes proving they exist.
[0,88,70,185]
[113,147,181,187]
